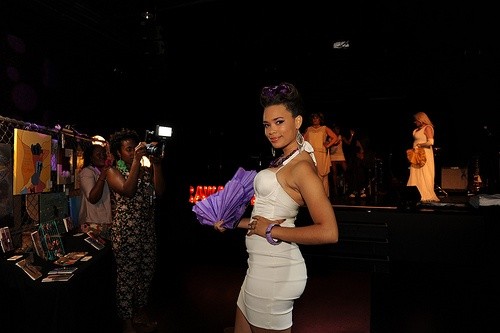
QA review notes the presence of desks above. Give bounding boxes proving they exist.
[0,227,108,333]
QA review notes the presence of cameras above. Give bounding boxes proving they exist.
[143,144,162,157]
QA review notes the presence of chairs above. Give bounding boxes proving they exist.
[369,274,492,333]
